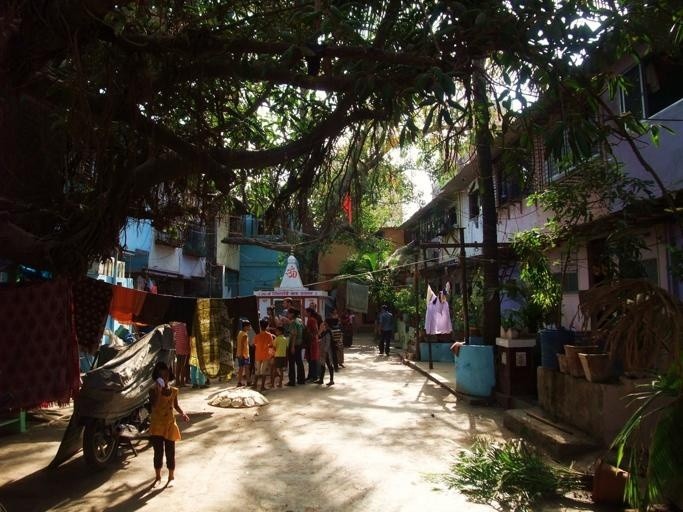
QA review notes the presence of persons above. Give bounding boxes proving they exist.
[499,278,524,320]
[172,296,356,392]
[147,361,190,489]
[374,304,393,356]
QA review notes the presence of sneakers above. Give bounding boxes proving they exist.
[192,384,209,388]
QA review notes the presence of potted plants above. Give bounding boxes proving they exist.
[448,436,631,512]
[393,288,481,343]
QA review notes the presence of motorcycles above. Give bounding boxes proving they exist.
[73,322,177,470]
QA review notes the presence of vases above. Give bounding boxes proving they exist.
[556,344,614,382]
